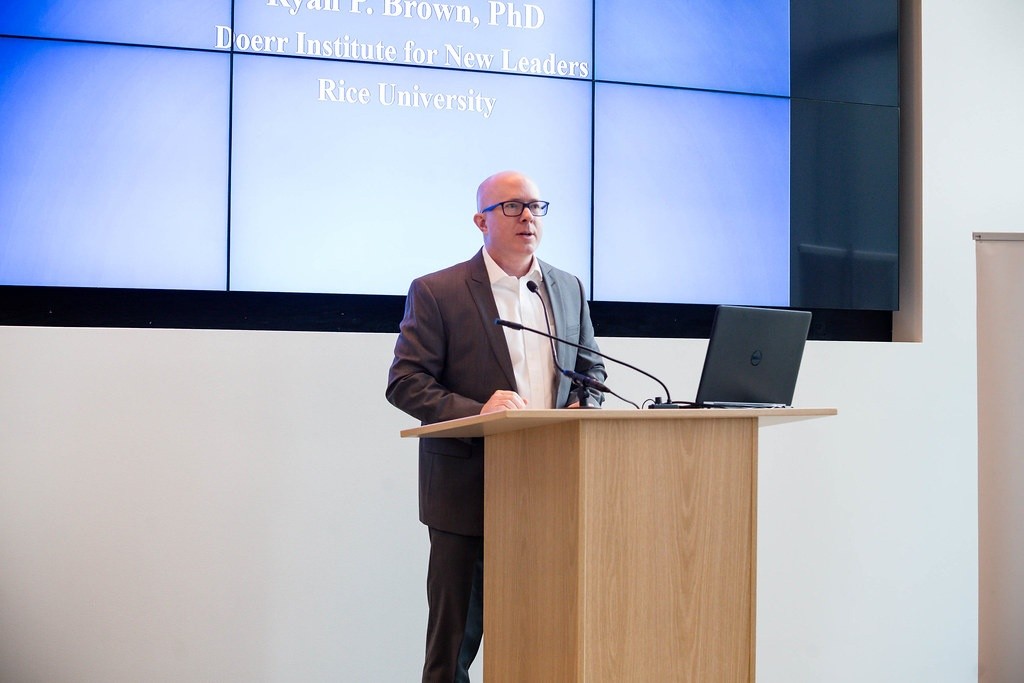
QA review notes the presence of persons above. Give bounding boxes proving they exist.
[385,170,608,683]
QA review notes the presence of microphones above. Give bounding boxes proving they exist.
[527,281,611,394]
[495,318,680,410]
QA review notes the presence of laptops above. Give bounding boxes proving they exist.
[679,305,812,409]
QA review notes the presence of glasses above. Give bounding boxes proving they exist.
[481,201,550,217]
